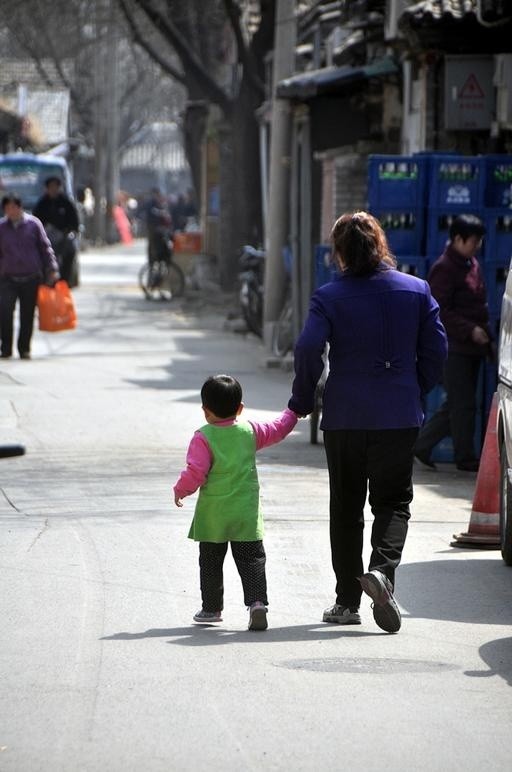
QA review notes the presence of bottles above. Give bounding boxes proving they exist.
[439,163,477,181]
[495,163,512,183]
[378,161,417,180]
[381,212,415,229]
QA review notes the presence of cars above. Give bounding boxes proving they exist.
[497,250,512,570]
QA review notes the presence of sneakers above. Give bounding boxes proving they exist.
[457,461,479,471]
[414,455,436,470]
[194,610,222,622]
[248,601,268,631]
[359,571,401,632]
[20,352,30,359]
[323,604,361,624]
[0,350,12,358]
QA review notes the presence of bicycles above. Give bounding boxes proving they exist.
[139,225,184,299]
[238,243,263,340]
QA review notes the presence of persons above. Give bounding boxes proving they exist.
[410,214,498,473]
[0,193,61,361]
[32,176,80,287]
[288,211,450,632]
[139,183,201,301]
[172,374,298,631]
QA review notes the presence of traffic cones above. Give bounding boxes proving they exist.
[452,388,509,554]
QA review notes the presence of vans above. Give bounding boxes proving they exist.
[1,154,75,220]
